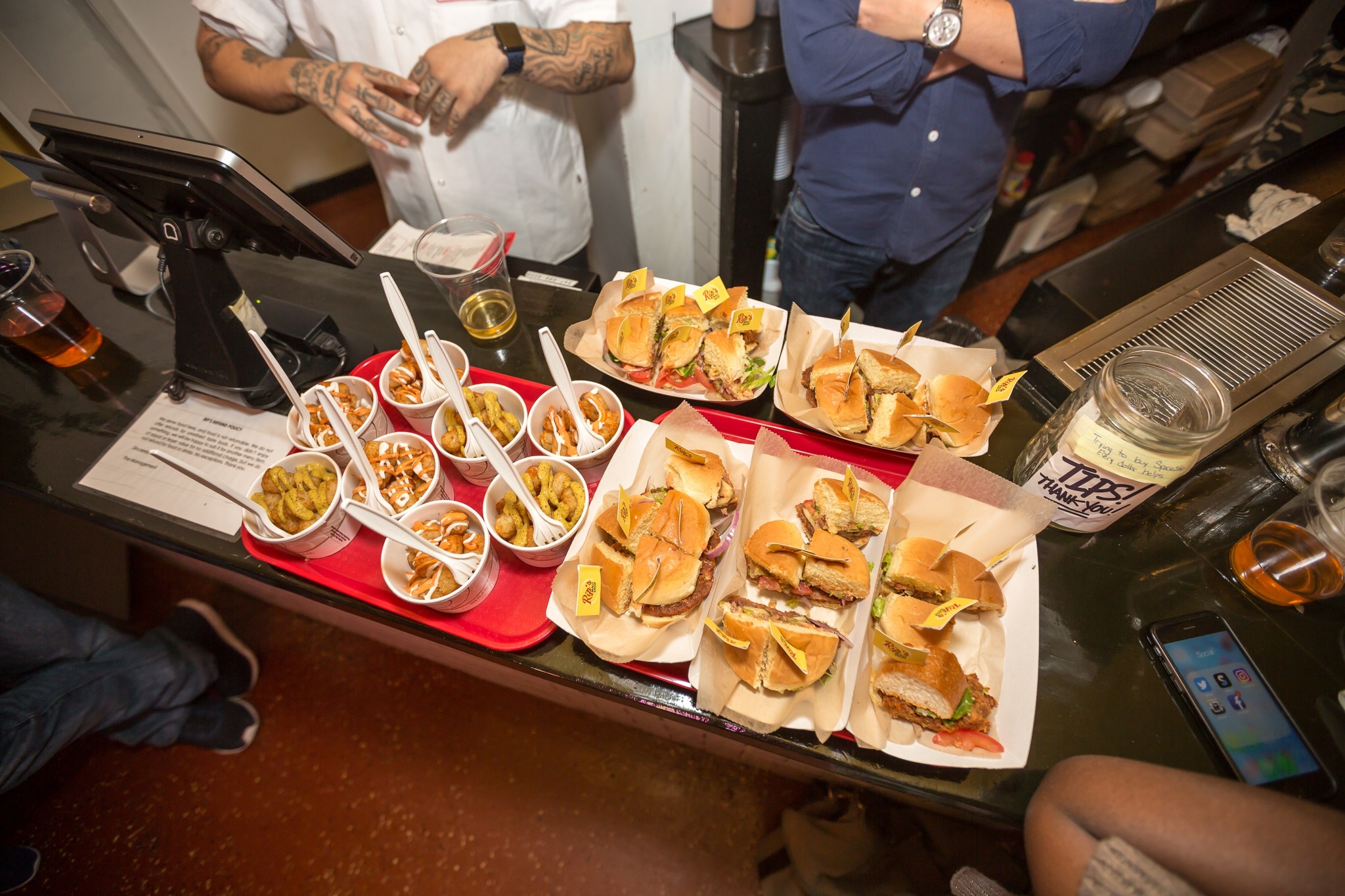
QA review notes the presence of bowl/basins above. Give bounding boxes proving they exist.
[339,432,454,520]
[527,380,626,484]
[287,376,399,468]
[241,450,363,558]
[431,383,530,487]
[381,500,501,614]
[482,455,590,568]
[379,341,473,435]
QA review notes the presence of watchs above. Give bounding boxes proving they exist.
[493,20,529,78]
[922,0,964,55]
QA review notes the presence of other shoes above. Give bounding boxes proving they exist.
[950,865,1015,896]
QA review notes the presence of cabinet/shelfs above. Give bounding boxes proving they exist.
[673,1,1345,305]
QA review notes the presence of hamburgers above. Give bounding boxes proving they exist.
[715,477,890,696]
[590,448,739,631]
[871,536,1006,753]
[800,339,995,449]
[600,284,768,401]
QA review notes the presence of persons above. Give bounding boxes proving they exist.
[768,0,1164,330]
[187,0,641,274]
[1022,754,1345,896]
[1,564,263,810]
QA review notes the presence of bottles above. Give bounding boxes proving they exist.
[1012,345,1231,532]
[998,153,1034,205]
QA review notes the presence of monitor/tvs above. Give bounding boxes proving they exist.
[26,106,364,268]
[2,150,153,244]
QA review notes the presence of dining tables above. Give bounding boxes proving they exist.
[0,195,1345,873]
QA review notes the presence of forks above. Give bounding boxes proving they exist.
[424,329,494,459]
[339,497,482,587]
[149,448,293,540]
[316,386,399,517]
[246,330,321,448]
[538,326,606,458]
[380,271,449,403]
[468,416,568,547]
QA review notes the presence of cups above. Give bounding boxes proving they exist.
[1,249,103,368]
[413,215,518,340]
[1229,458,1345,604]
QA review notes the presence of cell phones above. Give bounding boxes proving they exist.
[1145,610,1338,804]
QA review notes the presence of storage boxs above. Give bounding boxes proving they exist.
[1134,42,1276,163]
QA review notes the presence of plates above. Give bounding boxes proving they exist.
[774,90,808,182]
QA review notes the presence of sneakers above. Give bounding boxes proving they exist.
[174,697,260,755]
[168,598,258,698]
